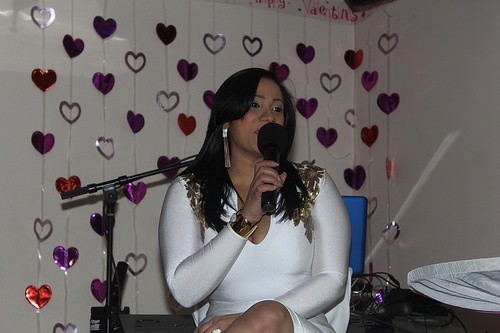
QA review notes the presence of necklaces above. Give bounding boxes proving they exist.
[229,179,245,206]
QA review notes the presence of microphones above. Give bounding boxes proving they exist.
[256,122,288,215]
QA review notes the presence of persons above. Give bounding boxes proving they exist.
[157,68,351,333]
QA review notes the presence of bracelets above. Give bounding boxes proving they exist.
[229,209,258,238]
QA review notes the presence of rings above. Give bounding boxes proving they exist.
[212,329,221,333]
